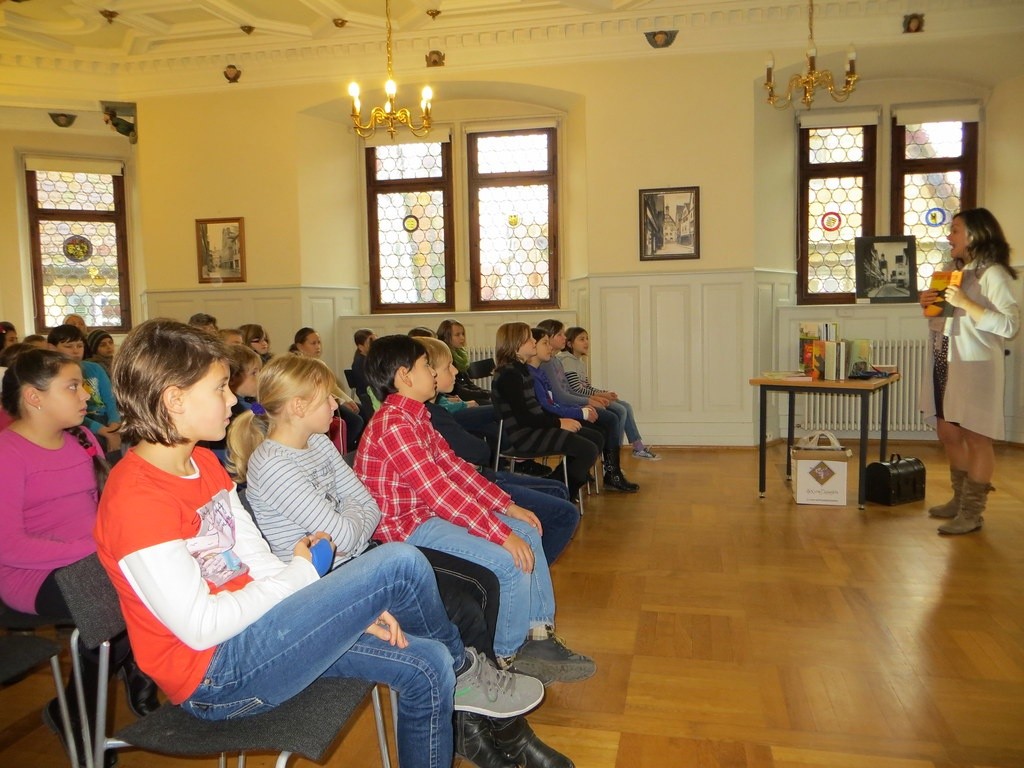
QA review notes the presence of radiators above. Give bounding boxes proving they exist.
[464,347,503,389]
[796,339,938,432]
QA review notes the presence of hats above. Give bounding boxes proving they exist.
[85,329,112,354]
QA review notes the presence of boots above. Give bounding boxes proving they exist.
[603,445,639,493]
[937,477,996,535]
[928,466,967,518]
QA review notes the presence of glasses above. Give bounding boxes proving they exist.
[250,339,266,344]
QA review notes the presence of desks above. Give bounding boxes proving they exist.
[749,373,901,510]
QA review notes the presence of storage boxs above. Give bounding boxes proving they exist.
[862,454,927,506]
[790,445,851,505]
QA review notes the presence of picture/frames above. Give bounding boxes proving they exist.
[639,186,699,259]
[195,216,246,282]
[855,236,918,304]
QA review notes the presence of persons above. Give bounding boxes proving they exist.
[94,319,544,768]
[411,336,581,569]
[0,314,132,469]
[920,208,1019,535]
[187,313,363,493]
[350,320,662,567]
[0,349,162,768]
[223,356,575,768]
[352,335,596,687]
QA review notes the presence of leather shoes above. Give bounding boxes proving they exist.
[451,712,517,768]
[489,716,575,768]
[41,696,117,768]
[115,651,164,718]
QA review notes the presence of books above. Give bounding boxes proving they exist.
[799,322,873,380]
[759,371,812,382]
[923,271,964,317]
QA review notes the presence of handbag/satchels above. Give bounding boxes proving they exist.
[790,429,853,506]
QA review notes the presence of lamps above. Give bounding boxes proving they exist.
[764,0,858,110]
[346,0,434,138]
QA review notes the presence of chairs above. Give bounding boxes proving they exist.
[0,405,604,768]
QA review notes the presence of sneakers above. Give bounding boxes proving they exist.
[496,657,554,688]
[450,646,545,718]
[631,446,662,462]
[514,459,552,478]
[513,635,596,683]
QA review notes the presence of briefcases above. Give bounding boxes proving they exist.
[864,453,927,505]
